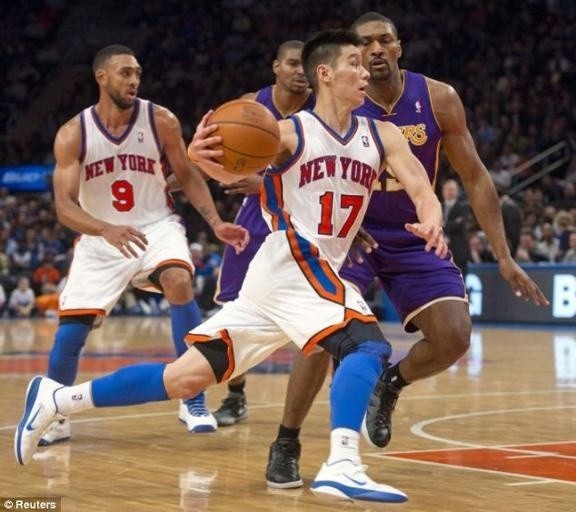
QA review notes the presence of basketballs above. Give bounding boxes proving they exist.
[204,100,280,175]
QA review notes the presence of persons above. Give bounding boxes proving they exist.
[0,0,576,506]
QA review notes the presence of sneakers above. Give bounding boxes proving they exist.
[265,440,304,489]
[38,418,71,446]
[178,390,217,433]
[14,375,69,467]
[211,391,249,427]
[361,368,399,450]
[309,458,409,506]
